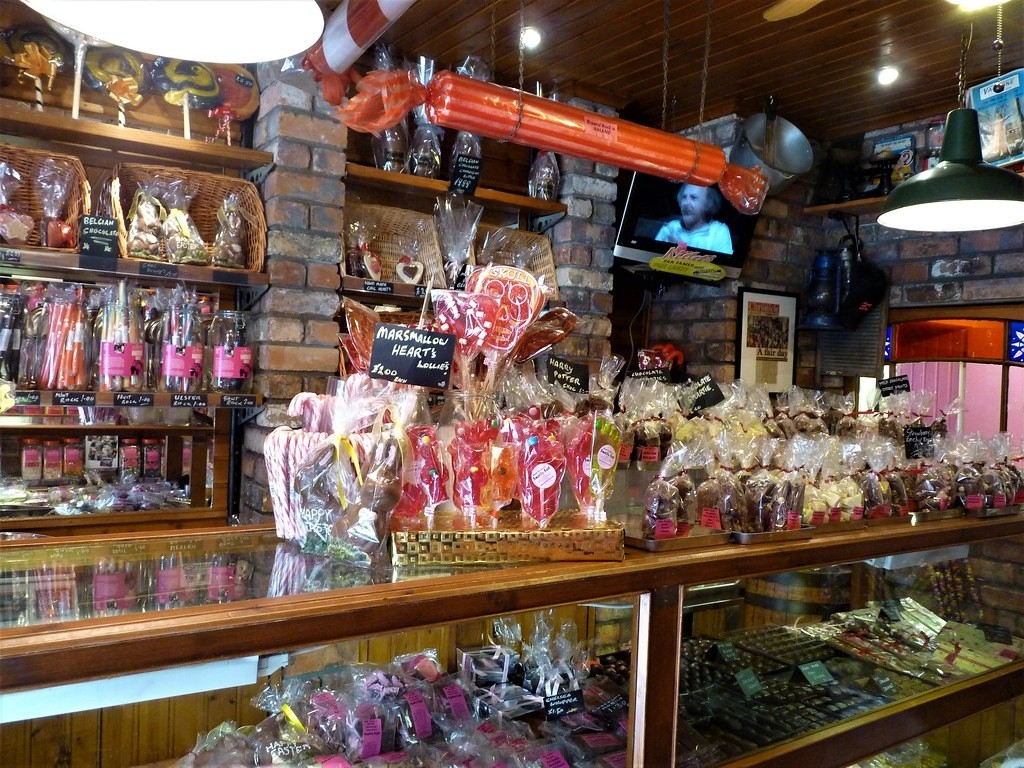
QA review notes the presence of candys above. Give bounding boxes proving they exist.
[336,246,1024,540]
[0,23,261,146]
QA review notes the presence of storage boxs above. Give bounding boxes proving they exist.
[967,68,1024,168]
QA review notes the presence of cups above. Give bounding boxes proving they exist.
[807,254,843,330]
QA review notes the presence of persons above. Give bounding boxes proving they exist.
[988,113,1012,156]
[655,183,735,255]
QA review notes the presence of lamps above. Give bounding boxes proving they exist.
[876,20,1024,232]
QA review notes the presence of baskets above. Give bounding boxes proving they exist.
[1,140,91,252]
[95,161,266,272]
[462,221,568,304]
[339,202,451,291]
[389,523,631,571]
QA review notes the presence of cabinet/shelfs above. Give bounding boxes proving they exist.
[0,523,1024,767]
[0,0,568,540]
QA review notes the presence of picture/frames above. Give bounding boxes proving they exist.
[734,285,801,398]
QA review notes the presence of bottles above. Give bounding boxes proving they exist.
[840,248,853,294]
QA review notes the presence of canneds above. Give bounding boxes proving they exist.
[20,437,167,480]
